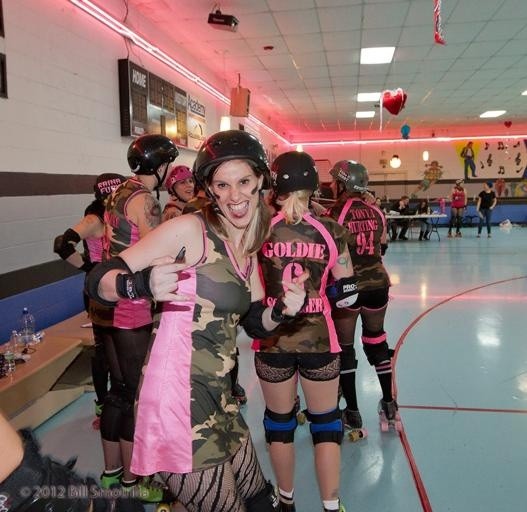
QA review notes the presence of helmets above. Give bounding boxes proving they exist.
[456,180,464,185]
[329,160,369,193]
[193,130,272,190]
[126,134,179,175]
[166,165,193,195]
[272,151,319,195]
[94,173,126,202]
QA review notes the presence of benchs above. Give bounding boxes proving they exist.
[0,336,85,433]
[40,309,95,391]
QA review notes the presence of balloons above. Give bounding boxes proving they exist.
[504,121,512,128]
[382,87,408,115]
[400,125,410,139]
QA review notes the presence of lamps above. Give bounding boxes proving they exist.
[390,154,401,168]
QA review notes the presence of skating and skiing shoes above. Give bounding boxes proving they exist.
[92,399,104,430]
[448,232,453,238]
[233,384,247,405]
[101,466,182,512]
[455,231,462,237]
[295,388,368,442]
[378,399,402,433]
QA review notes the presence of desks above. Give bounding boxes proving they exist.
[385,212,447,241]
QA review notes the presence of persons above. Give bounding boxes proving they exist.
[476,179,498,239]
[461,141,478,179]
[411,160,444,195]
[0,405,146,512]
[52,128,398,512]
[414,199,433,240]
[390,195,413,240]
[447,179,468,237]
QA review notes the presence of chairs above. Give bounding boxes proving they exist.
[388,219,422,241]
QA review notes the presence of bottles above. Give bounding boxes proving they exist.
[3,307,37,376]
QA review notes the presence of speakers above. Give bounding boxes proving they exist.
[229,87,251,117]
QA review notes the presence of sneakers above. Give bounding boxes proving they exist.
[399,235,408,240]
[392,233,397,240]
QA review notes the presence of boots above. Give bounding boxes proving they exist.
[424,231,429,239]
[419,231,423,240]
